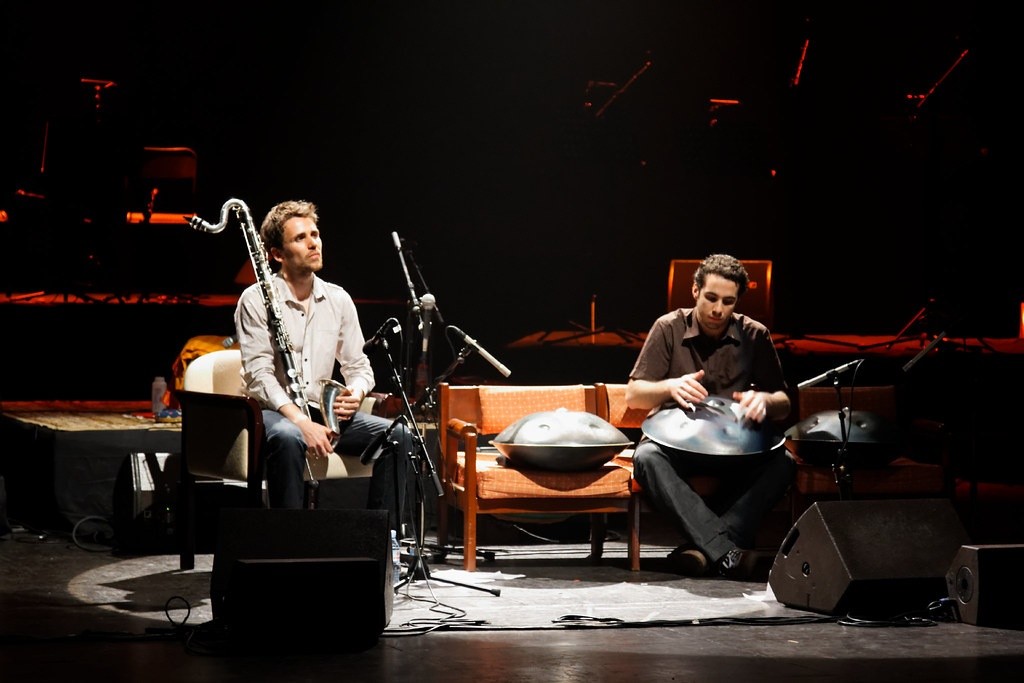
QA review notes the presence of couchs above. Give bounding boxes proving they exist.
[174,347,389,571]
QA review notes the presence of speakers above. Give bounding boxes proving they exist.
[666,259,776,331]
[945,544,1024,625]
[768,499,971,614]
[210,510,396,630]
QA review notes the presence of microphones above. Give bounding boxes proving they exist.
[421,294,435,361]
[359,416,402,465]
[797,358,862,391]
[362,320,390,354]
[902,332,946,372]
[452,326,511,378]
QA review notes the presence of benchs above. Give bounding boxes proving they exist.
[439,381,951,574]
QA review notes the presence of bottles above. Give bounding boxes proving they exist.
[391,530,400,586]
[152,377,167,413]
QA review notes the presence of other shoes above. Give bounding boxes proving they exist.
[722,546,761,577]
[668,543,707,576]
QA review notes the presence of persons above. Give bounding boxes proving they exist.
[626,254,797,575]
[233,199,411,625]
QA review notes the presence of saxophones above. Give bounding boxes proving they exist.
[182,198,355,454]
